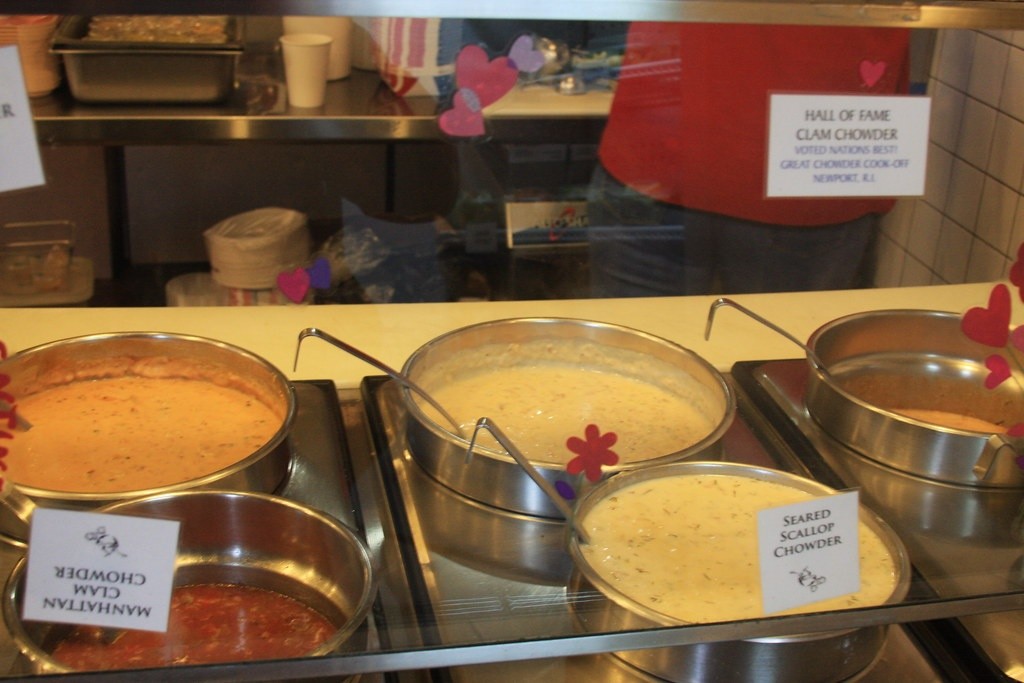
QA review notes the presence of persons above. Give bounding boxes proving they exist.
[586,0,913,295]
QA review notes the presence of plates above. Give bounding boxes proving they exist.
[1,15,60,97]
[204,209,312,290]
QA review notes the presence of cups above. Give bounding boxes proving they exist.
[280,34,334,108]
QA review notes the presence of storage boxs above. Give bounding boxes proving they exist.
[504,197,594,251]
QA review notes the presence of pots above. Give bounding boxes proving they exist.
[0,330,297,501]
[566,461,913,683]
[401,316,737,586]
[2,490,379,673]
[806,309,1024,546]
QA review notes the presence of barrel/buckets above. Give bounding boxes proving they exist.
[282,15,352,81]
